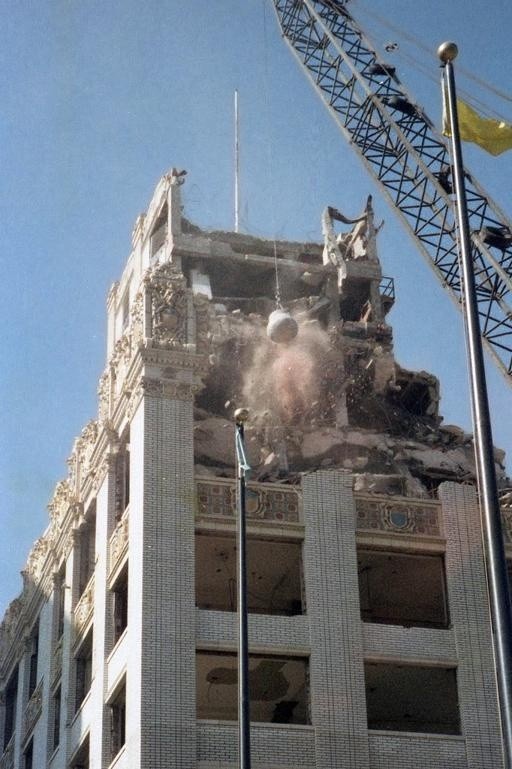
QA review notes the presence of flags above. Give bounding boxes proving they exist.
[441,78,512,157]
